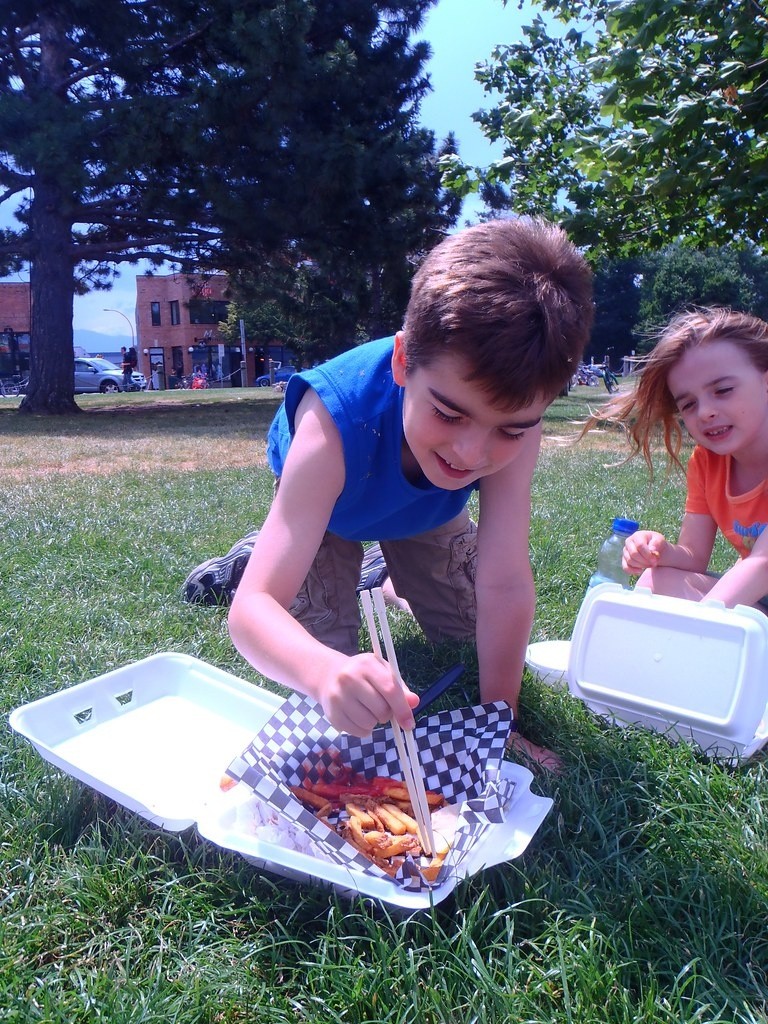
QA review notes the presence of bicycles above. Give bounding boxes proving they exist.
[571,365,598,389]
[1,373,30,399]
[599,357,620,395]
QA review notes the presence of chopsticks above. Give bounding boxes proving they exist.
[361,588,435,860]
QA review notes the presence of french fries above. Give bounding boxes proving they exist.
[290,783,443,858]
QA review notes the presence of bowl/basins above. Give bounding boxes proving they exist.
[525,641,570,691]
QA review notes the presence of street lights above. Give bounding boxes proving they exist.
[103,308,135,347]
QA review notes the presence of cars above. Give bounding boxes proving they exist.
[74,357,147,394]
[255,365,310,388]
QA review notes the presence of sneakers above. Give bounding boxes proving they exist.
[356,544,387,593]
[179,531,260,605]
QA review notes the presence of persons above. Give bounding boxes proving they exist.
[121,347,142,392]
[182,220,595,774]
[575,310,768,609]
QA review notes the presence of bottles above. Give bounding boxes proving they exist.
[585,518,639,595]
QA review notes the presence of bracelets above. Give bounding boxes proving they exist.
[509,719,519,732]
